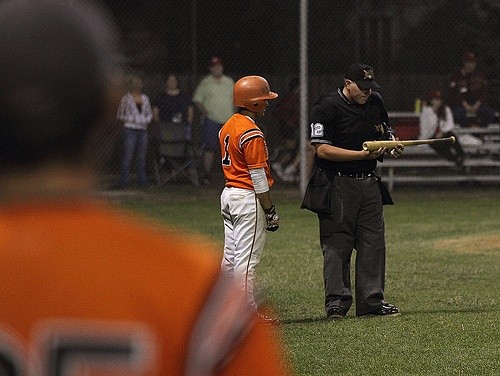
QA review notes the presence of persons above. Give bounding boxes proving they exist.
[0,6,284,376]
[221,75,281,328]
[192,56,238,187]
[446,50,493,129]
[117,76,154,193]
[419,90,471,174]
[154,73,194,184]
[308,63,406,319]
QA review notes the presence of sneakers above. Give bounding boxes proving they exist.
[255,312,279,325]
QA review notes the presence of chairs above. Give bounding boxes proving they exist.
[155,121,205,188]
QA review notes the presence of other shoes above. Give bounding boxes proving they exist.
[203,179,209,184]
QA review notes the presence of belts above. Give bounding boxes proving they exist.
[338,172,372,179]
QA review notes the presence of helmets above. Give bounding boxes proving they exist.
[234,76,278,112]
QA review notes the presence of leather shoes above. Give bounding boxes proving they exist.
[369,303,402,317]
[327,307,344,318]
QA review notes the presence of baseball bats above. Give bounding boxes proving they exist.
[362,135,456,152]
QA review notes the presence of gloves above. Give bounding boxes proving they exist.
[264,205,279,232]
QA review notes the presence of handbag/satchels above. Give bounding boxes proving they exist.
[372,171,394,205]
[299,164,332,213]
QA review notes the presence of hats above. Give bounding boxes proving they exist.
[464,51,476,60]
[432,88,444,98]
[210,57,223,65]
[345,63,380,90]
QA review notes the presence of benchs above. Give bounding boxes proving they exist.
[375,111,500,193]
